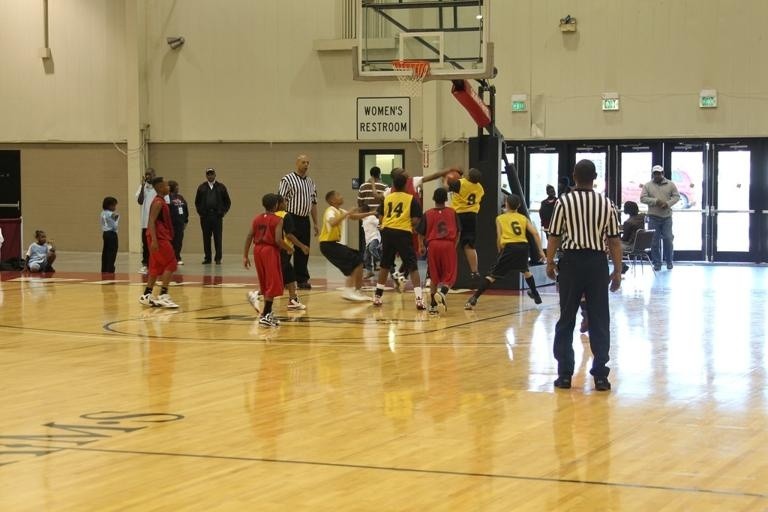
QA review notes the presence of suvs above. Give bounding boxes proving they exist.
[611,164,699,215]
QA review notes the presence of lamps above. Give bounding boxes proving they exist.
[560,18,576,24]
[167,36,185,50]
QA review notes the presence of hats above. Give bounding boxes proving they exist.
[651,164,665,172]
[206,168,215,173]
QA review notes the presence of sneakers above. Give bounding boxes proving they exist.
[653,261,673,270]
[527,291,542,305]
[414,297,427,310]
[258,315,280,326]
[554,373,572,389]
[288,300,306,310]
[464,298,479,310]
[373,295,383,306]
[138,257,224,275]
[156,293,181,310]
[392,272,408,294]
[248,289,262,314]
[341,290,373,303]
[434,292,448,314]
[425,276,432,289]
[428,305,439,316]
[468,272,482,279]
[592,375,612,390]
[137,293,162,308]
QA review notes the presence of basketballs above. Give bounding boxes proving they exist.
[444,170,462,186]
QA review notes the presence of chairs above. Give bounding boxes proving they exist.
[623,229,657,276]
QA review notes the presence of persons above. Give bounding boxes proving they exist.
[135,168,171,275]
[640,165,680,271]
[99,197,120,272]
[169,180,188,265]
[195,169,230,264]
[20,230,56,273]
[138,177,179,308]
